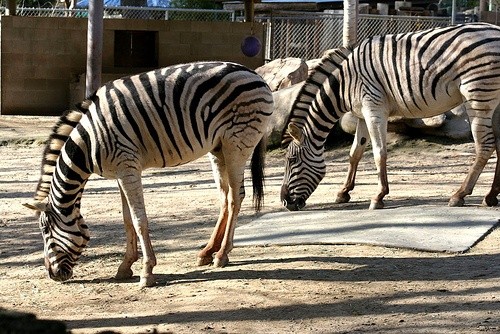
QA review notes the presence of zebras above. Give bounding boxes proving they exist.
[21,60,275,288]
[280,21,500,211]
